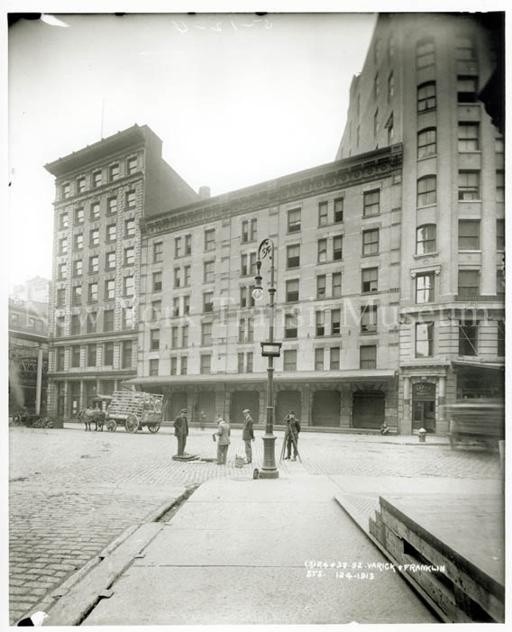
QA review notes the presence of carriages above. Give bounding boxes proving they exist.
[79,388,166,433]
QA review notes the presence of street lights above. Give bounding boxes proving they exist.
[248,235,281,482]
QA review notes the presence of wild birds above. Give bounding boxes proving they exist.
[17,611,50,626]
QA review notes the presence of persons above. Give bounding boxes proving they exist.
[283,410,301,461]
[242,410,254,463]
[174,409,188,456]
[213,418,230,465]
[198,411,207,430]
